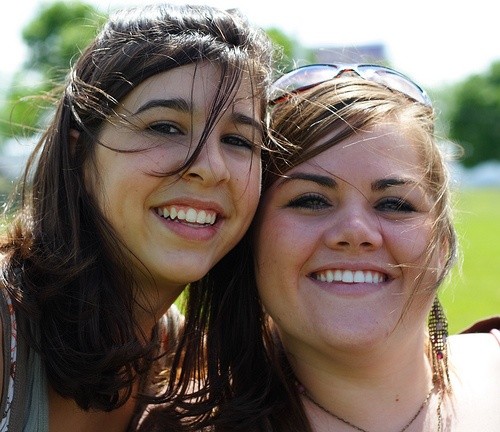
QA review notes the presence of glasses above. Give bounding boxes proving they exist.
[266,61,431,111]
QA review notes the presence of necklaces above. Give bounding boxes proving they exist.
[290,367,436,432]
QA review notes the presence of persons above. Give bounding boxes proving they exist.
[2,5,270,432]
[128,63,499,431]
